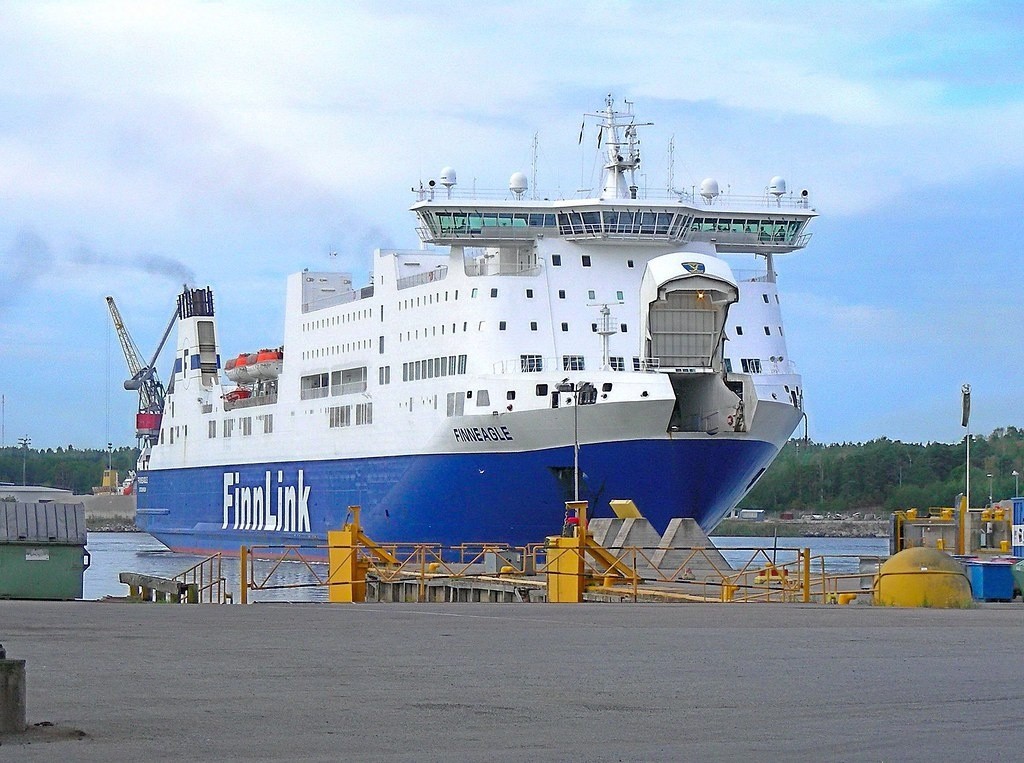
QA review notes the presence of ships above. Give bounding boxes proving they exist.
[100,92,816,567]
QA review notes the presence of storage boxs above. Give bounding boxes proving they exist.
[965,560,1015,602]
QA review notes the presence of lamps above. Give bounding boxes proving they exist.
[554,381,598,405]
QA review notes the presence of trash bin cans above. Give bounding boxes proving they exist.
[0,502,91,601]
[966,560,1014,603]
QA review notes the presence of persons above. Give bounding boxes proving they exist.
[744,226,751,233]
[774,225,786,241]
[758,227,771,236]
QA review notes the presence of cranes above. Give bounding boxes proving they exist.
[93,293,164,486]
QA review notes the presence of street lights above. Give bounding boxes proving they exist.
[1011,470,1020,498]
[986,472,993,503]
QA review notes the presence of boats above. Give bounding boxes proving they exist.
[225,385,252,402]
[244,353,261,378]
[224,358,242,381]
[256,348,284,378]
[235,353,256,382]
